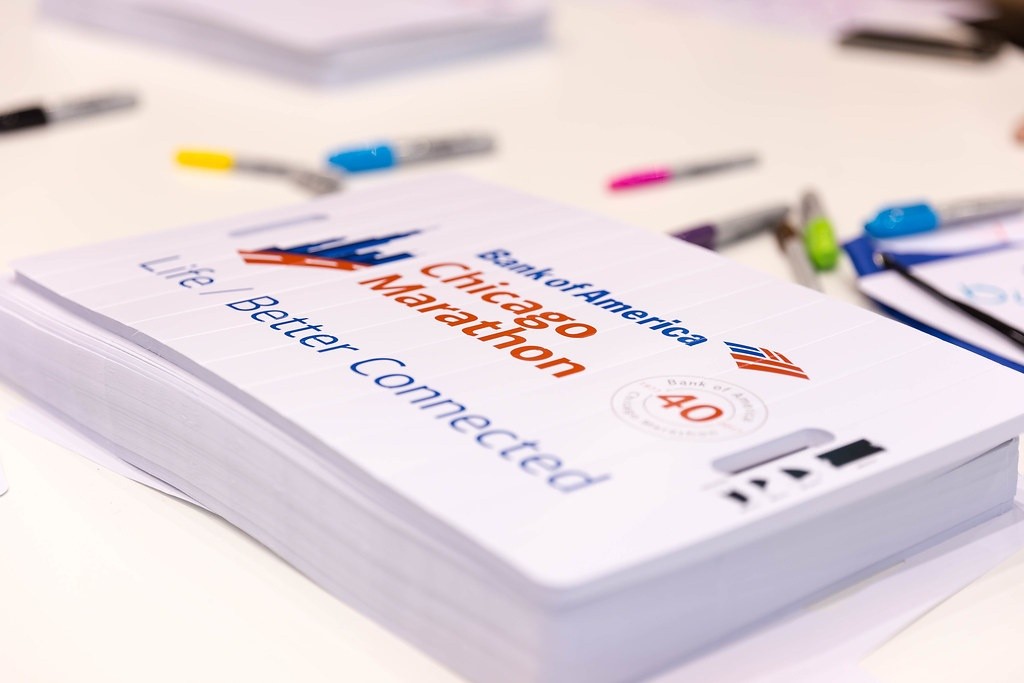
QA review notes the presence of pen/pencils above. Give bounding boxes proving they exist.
[606,149,758,193]
[166,147,345,196]
[771,184,838,292]
[667,201,787,251]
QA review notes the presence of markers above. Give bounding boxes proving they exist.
[0,84,142,137]
[323,127,495,175]
[857,189,1024,243]
